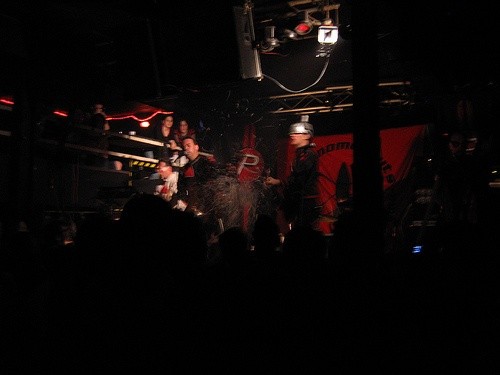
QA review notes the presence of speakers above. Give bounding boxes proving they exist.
[75,166,128,212]
[127,160,158,191]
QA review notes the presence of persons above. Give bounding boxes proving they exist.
[172,117,198,170]
[80,102,110,168]
[143,114,176,170]
[0,139,500,375]
[170,135,215,227]
[264,110,320,242]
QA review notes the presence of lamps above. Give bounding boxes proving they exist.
[294,11,312,35]
[273,16,297,40]
[252,22,277,51]
[318,10,339,58]
